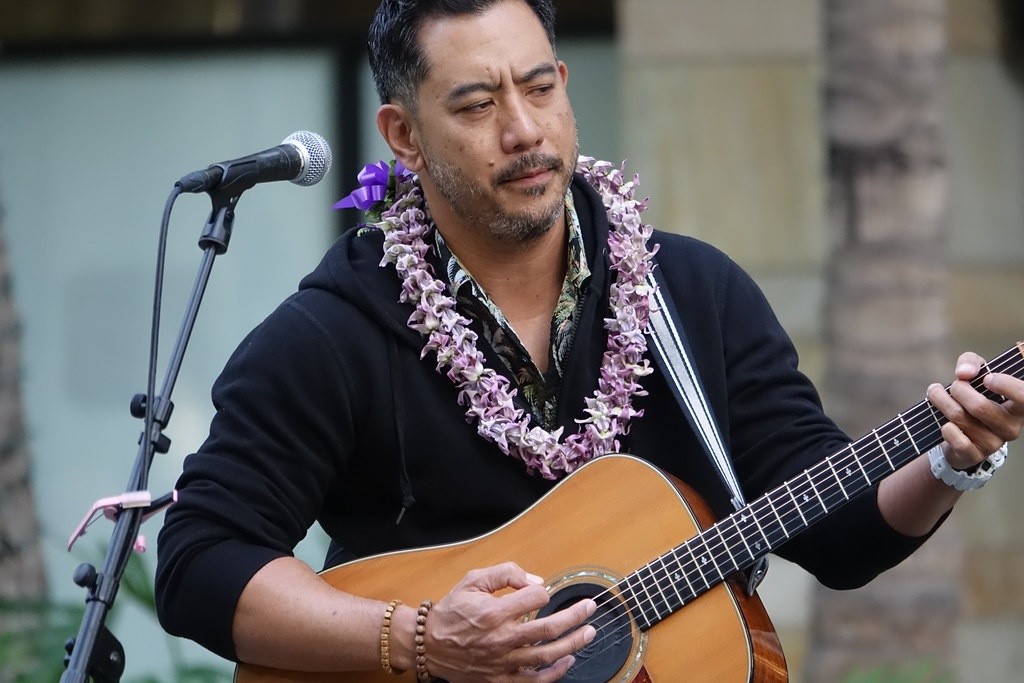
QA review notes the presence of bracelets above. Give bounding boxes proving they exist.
[414,599,434,683]
[379,598,407,678]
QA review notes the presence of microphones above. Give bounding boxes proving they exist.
[175,131,332,194]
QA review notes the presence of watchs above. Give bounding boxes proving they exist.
[926,440,1010,491]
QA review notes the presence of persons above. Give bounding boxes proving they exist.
[154,0,1024,683]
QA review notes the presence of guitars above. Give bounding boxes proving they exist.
[229,339,1024,683]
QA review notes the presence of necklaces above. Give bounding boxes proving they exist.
[356,153,659,483]
